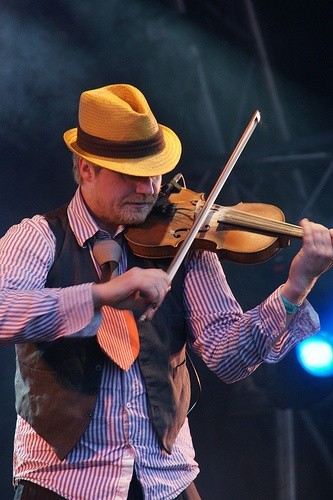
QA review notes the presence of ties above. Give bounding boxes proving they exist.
[90,236,140,371]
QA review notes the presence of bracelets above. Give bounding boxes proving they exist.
[280,292,304,315]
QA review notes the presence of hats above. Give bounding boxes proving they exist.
[63,84,182,176]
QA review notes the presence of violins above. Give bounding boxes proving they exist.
[122,184,333,259]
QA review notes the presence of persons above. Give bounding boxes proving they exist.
[0,83,333,500]
